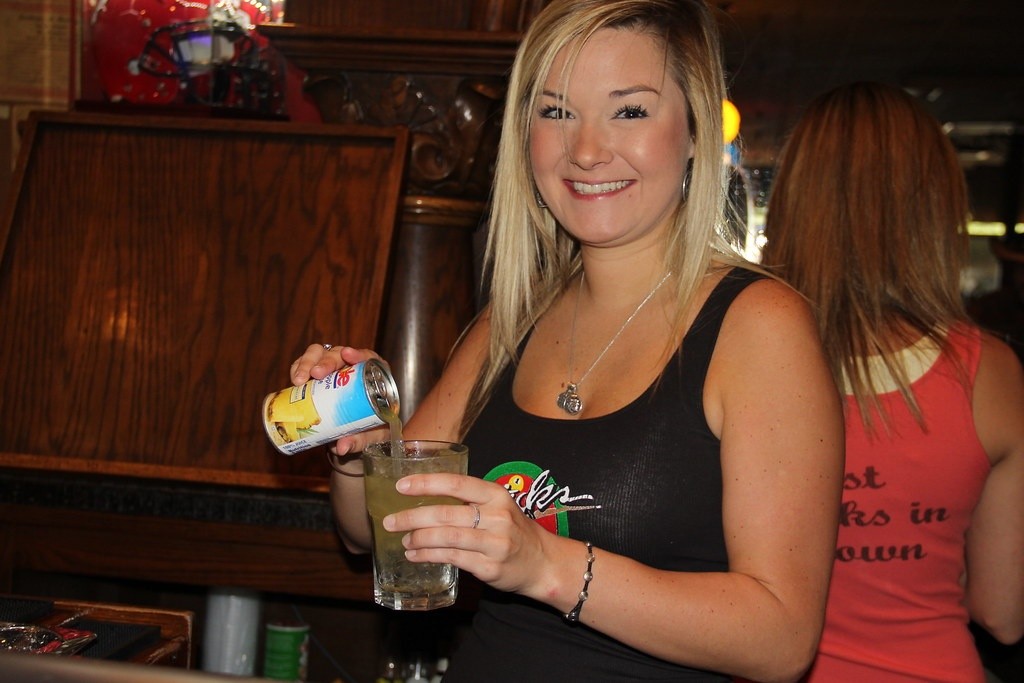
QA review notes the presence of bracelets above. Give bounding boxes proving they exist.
[563,541,596,622]
[326,448,364,477]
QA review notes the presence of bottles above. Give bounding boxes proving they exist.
[375,652,429,683]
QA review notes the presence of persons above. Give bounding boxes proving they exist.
[758,81,1024,683]
[285,0,846,683]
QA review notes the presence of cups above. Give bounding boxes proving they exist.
[202,585,262,677]
[264,623,311,682]
[363,440,468,610]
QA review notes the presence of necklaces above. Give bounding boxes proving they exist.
[557,271,673,416]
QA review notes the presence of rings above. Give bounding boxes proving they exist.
[323,344,332,350]
[469,503,480,529]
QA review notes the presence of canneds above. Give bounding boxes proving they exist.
[261,357,401,456]
[263,622,310,682]
